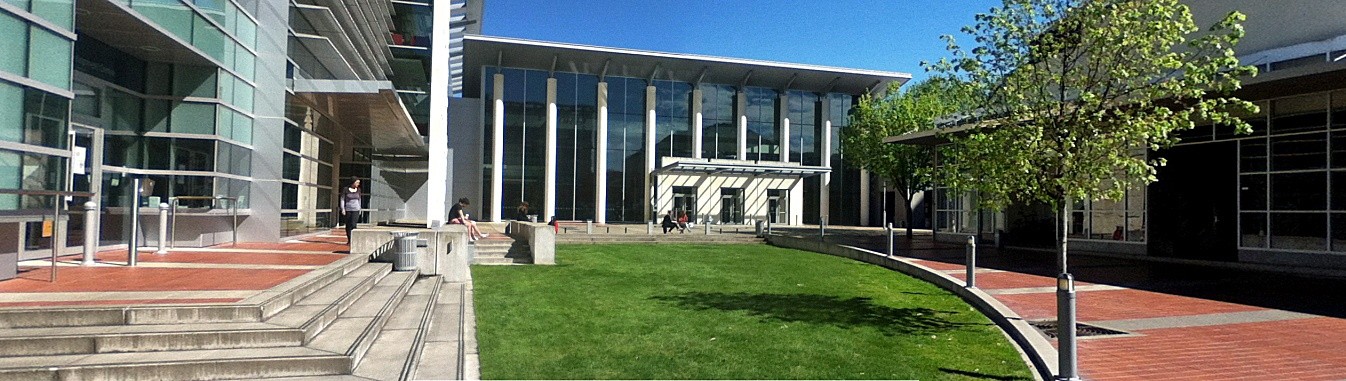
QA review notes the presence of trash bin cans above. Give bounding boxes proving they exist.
[755,219,764,237]
[390,231,419,271]
[526,214,538,223]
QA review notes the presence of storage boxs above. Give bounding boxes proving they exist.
[139,196,160,208]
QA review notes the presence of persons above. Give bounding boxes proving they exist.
[677,213,691,234]
[448,197,489,243]
[663,210,683,234]
[517,202,532,222]
[340,175,362,246]
[548,216,559,234]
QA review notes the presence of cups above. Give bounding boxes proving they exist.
[465,213,470,220]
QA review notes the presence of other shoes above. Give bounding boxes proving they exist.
[469,240,474,243]
[688,230,691,232]
[481,234,489,239]
[677,228,683,230]
[473,237,478,240]
[347,243,350,245]
